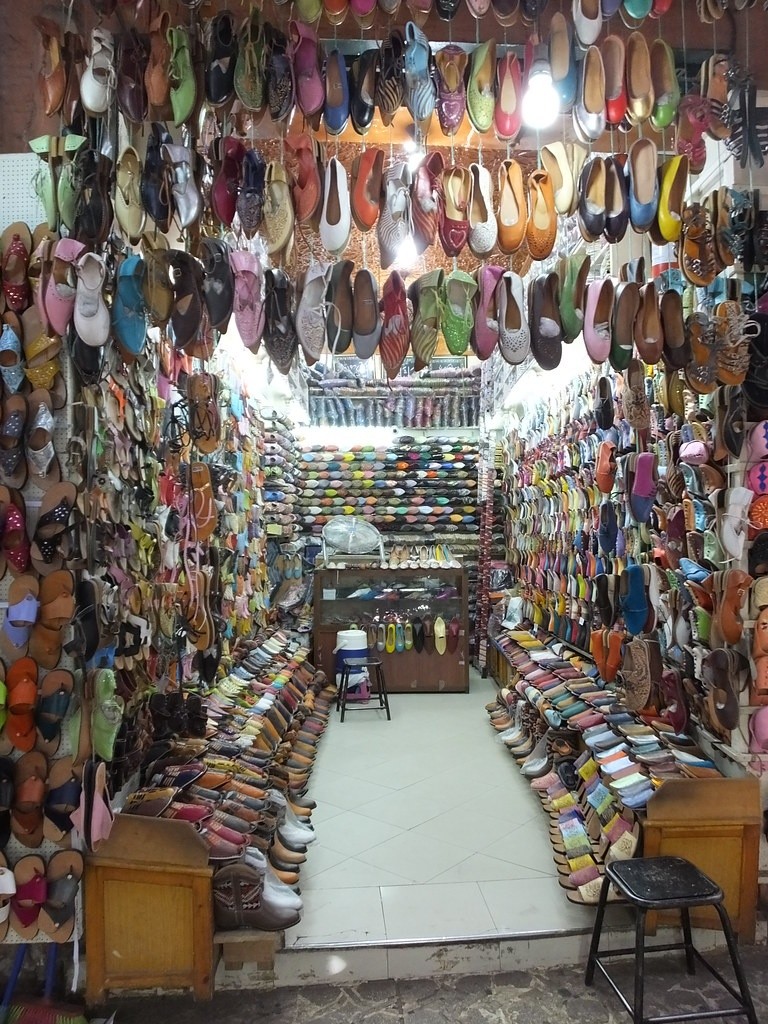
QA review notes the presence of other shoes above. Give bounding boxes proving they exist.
[33,0,768,934]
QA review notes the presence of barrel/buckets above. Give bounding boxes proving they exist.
[332,629,369,673]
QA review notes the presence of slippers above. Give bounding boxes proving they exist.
[0,219,66,946]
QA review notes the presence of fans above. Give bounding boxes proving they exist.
[321,517,385,565]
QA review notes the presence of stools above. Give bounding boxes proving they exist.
[584,857,758,1024]
[336,656,391,722]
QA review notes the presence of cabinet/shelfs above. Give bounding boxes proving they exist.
[313,554,469,695]
